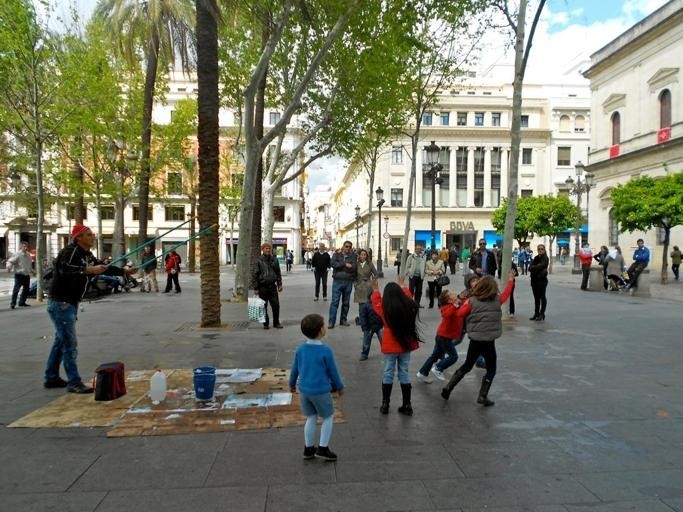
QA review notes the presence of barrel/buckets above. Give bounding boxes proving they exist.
[192,366,216,376]
[194,374,215,401]
[150,369,167,401]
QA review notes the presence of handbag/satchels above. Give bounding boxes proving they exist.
[248,295,266,323]
[437,270,450,286]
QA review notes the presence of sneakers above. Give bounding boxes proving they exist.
[44,376,94,394]
[304,445,337,460]
[314,297,327,301]
[417,367,445,383]
[361,357,368,361]
[328,320,349,329]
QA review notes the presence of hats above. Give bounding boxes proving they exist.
[72,225,89,239]
[262,243,271,249]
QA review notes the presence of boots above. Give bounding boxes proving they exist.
[380,381,393,414]
[399,383,413,416]
[477,376,494,406]
[441,371,464,400]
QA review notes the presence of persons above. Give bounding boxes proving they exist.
[42,223,139,394]
[577,239,650,296]
[6,240,50,309]
[288,313,345,461]
[96,244,183,294]
[368,275,425,416]
[394,238,534,384]
[560,246,567,267]
[440,268,516,406]
[527,244,549,321]
[286,240,389,361]
[670,246,682,280]
[250,241,285,329]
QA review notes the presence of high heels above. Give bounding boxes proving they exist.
[530,313,545,321]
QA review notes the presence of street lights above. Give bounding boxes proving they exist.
[355,206,359,252]
[571,160,584,275]
[105,125,139,268]
[376,187,384,278]
[424,141,440,249]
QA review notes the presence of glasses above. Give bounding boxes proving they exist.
[538,248,543,250]
[346,246,352,248]
[479,243,485,245]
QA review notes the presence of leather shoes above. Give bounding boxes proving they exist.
[11,303,30,308]
[264,324,283,329]
